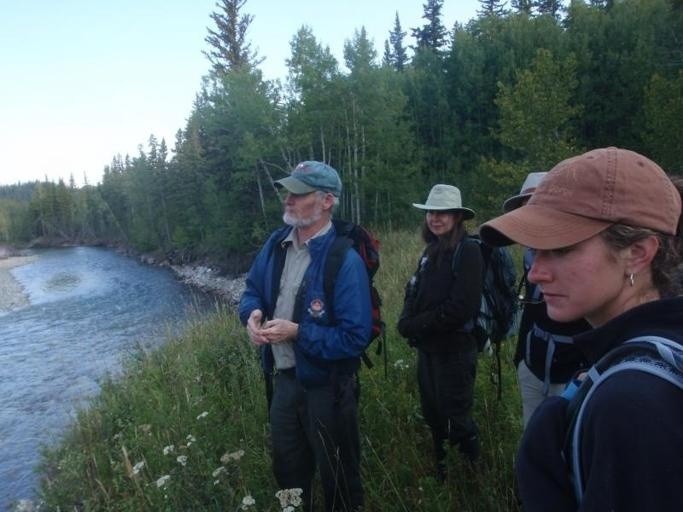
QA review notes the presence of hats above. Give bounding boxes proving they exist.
[274,161,343,195]
[478,146,683,251]
[503,171,550,214]
[412,184,476,220]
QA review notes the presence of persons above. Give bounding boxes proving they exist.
[514,145,683,512]
[504,171,597,422]
[398,182,493,484]
[238,160,373,512]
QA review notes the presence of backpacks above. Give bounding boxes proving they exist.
[331,219,382,369]
[464,231,526,352]
